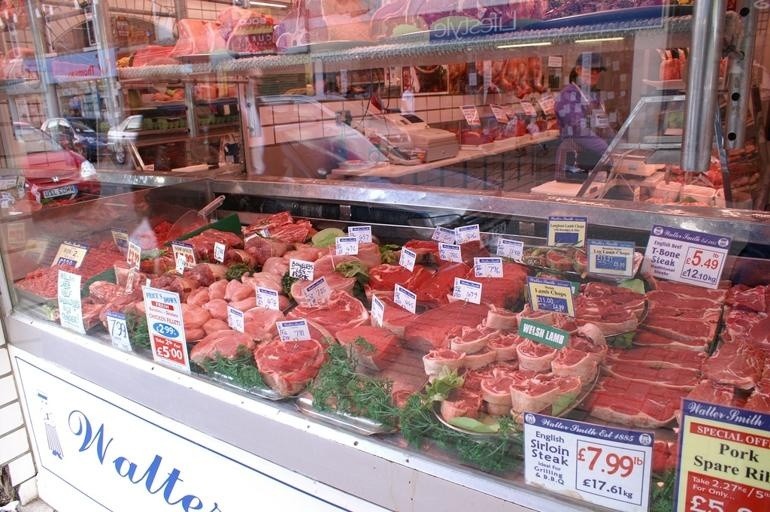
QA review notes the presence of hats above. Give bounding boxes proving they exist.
[576,52,608,72]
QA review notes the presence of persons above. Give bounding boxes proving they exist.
[554,52,615,184]
